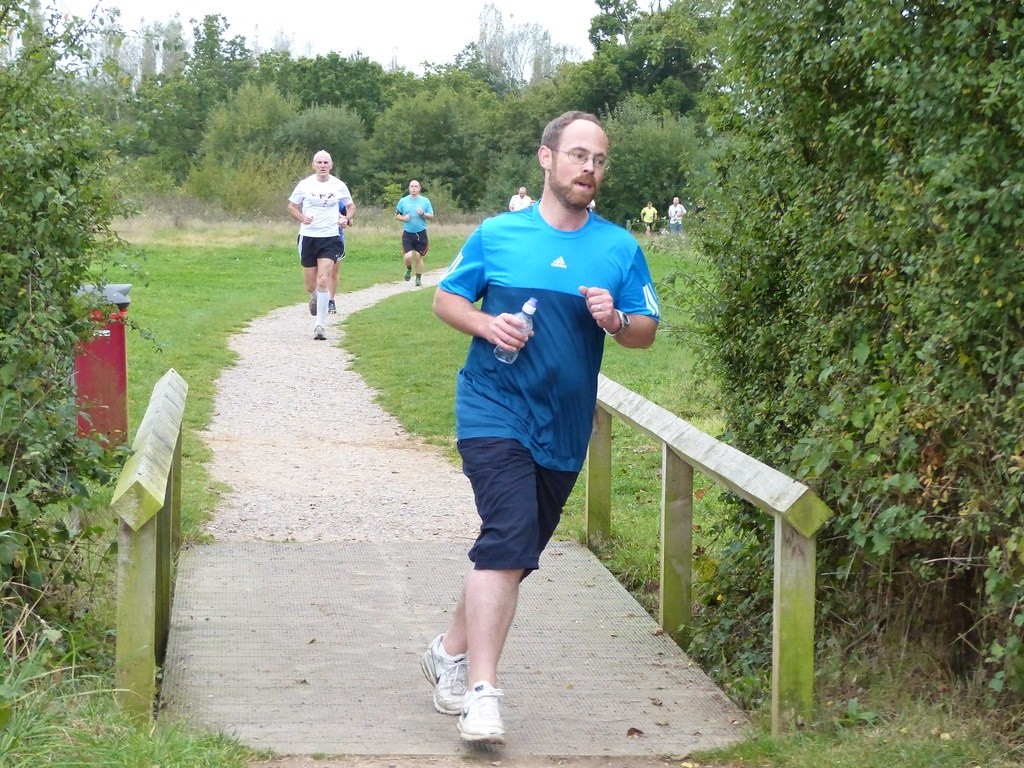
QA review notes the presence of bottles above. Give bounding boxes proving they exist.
[493,297,537,364]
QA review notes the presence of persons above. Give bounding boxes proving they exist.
[585,198,597,213]
[326,173,353,314]
[668,197,687,238]
[418,109,660,746]
[509,187,532,214]
[287,149,357,341]
[641,201,658,239]
[395,179,434,287]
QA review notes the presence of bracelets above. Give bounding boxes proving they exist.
[421,211,425,216]
[345,216,350,226]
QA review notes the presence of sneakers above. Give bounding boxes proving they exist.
[456,682,506,747]
[328,300,336,314]
[415,273,422,286]
[405,266,412,281]
[310,299,318,316]
[421,634,470,714]
[314,324,326,340]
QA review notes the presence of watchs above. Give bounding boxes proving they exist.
[602,308,630,337]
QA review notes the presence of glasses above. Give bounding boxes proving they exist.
[549,148,611,171]
